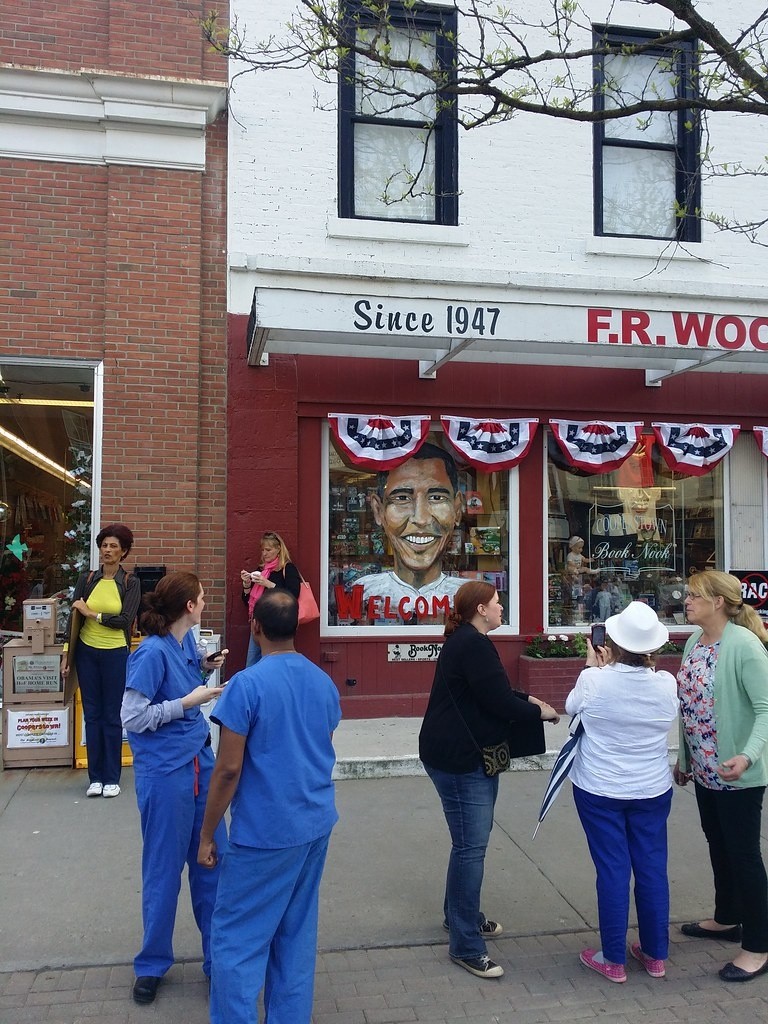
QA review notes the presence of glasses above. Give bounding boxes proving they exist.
[685,591,725,603]
[264,532,280,545]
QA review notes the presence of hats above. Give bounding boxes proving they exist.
[605,601,669,654]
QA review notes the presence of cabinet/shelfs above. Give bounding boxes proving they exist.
[329,508,510,595]
[660,516,716,567]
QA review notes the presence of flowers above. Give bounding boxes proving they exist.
[524,626,684,658]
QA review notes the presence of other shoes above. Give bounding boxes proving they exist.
[630,943,665,977]
[580,949,626,983]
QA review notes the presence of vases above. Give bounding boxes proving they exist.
[518,653,683,714]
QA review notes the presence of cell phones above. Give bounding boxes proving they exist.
[591,625,606,652]
[206,652,222,662]
[215,680,229,688]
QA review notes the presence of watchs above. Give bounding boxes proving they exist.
[742,754,753,771]
[96,612,102,624]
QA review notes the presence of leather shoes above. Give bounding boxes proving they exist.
[718,957,768,982]
[133,976,161,1002]
[681,922,742,943]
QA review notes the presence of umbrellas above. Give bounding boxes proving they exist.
[531,712,584,842]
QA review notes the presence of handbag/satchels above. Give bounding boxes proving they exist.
[485,739,510,777]
[283,560,320,627]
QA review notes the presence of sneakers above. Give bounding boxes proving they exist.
[87,782,103,796]
[450,953,504,978]
[442,915,503,937]
[102,784,120,797]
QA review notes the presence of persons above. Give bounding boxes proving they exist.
[239,531,302,670]
[593,583,612,620]
[59,524,141,798]
[418,582,561,977]
[567,535,601,574]
[676,570,768,982]
[119,572,229,1005]
[197,589,341,1024]
[564,602,679,984]
